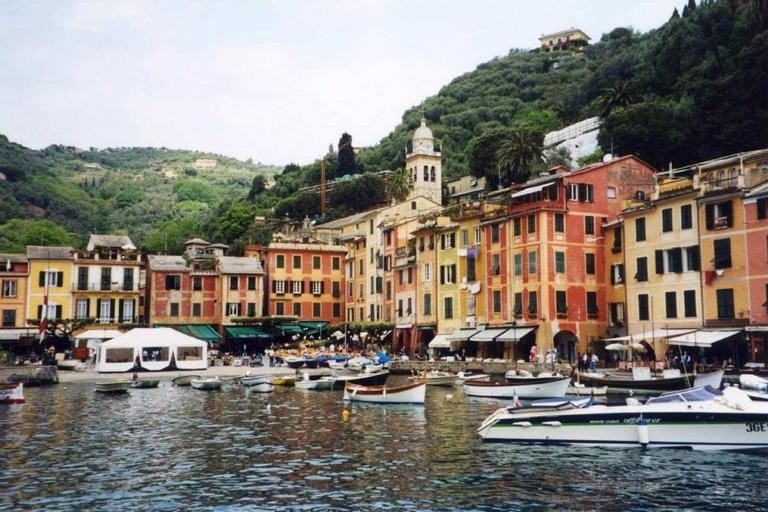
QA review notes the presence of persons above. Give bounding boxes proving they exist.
[528,345,735,374]
[31,345,58,366]
[192,341,466,365]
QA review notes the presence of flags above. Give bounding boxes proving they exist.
[37,268,49,342]
[459,247,477,257]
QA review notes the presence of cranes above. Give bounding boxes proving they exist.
[318,139,353,217]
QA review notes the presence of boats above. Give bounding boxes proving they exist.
[476,384,768,450]
[477,435,767,508]
[407,366,460,387]
[343,400,426,428]
[95,321,390,392]
[504,368,565,383]
[0,382,25,404]
[343,369,426,405]
[462,343,573,401]
[566,384,608,396]
[578,297,768,400]
[457,367,490,382]
